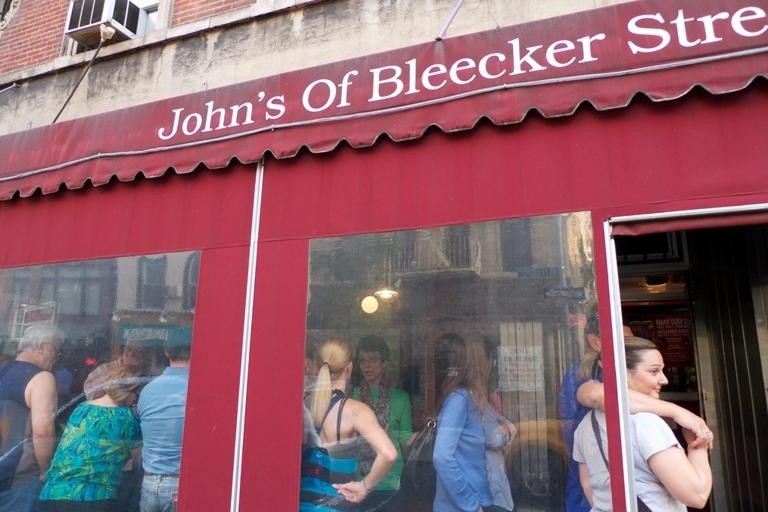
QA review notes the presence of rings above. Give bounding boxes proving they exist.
[705,427,710,434]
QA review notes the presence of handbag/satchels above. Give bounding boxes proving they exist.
[399,416,437,503]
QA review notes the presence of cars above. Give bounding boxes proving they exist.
[491,411,585,509]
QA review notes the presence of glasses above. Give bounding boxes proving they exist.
[356,356,382,365]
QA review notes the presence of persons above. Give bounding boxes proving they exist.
[432,325,494,511]
[298,338,397,511]
[556,312,715,512]
[345,334,420,510]
[0,312,194,511]
[573,335,712,512]
[469,334,517,511]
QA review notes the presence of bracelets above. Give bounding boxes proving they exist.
[361,479,374,496]
